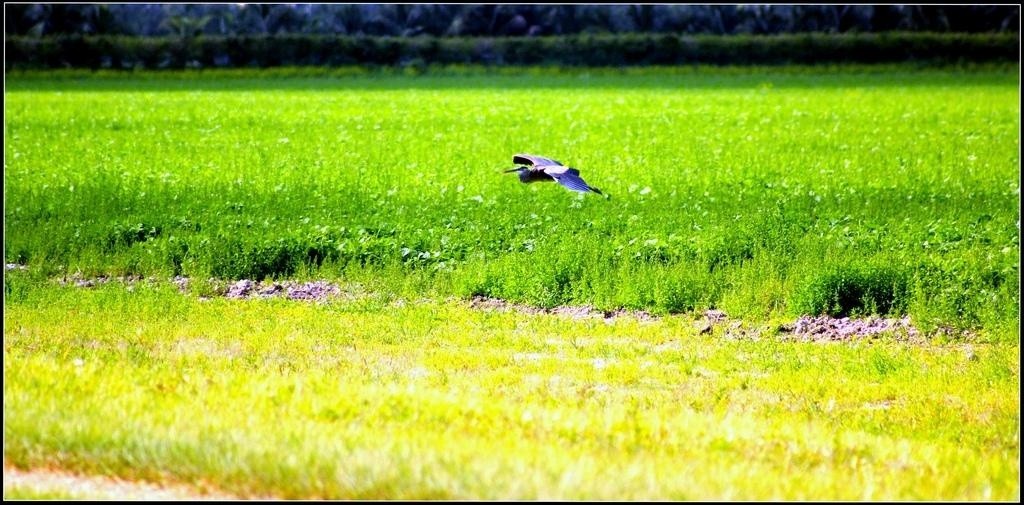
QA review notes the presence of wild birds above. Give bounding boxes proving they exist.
[503,153,603,194]
